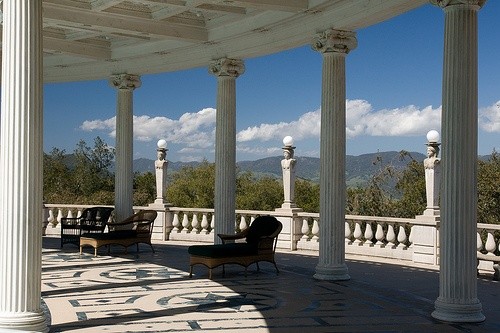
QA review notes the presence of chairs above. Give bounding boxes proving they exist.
[188,216,283,280]
[61,206,115,251]
[80,210,157,258]
